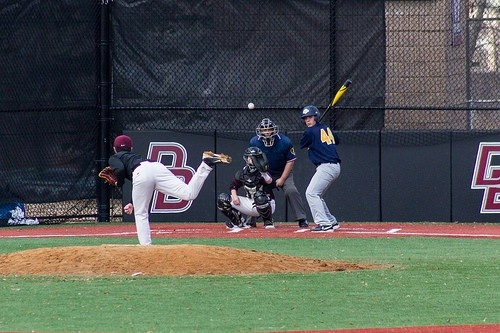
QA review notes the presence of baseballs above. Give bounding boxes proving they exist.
[248,103,254,110]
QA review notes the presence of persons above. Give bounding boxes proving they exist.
[250,118,309,228]
[98,135,232,245]
[299,105,342,233]
[218,147,276,228]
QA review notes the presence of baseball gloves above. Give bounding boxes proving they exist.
[251,151,269,172]
[99,166,118,187]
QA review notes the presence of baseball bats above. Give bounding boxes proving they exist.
[317,79,352,122]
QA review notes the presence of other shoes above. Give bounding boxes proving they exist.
[247,221,256,227]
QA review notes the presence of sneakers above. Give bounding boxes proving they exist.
[331,222,340,230]
[299,219,308,227]
[202,151,232,166]
[310,224,334,233]
[226,217,251,229]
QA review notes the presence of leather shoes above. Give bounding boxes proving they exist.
[263,218,274,229]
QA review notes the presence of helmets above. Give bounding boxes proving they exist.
[300,105,320,121]
[113,135,133,151]
[255,118,279,147]
[243,147,262,168]
